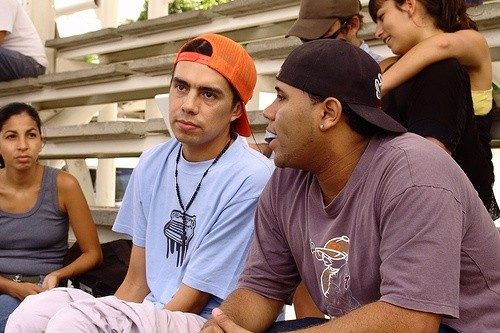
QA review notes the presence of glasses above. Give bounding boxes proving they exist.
[299,23,350,43]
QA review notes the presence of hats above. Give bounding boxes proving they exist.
[174,34,258,137]
[285,0,360,40]
[276,38,408,134]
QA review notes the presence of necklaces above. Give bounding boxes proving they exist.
[175,139,233,250]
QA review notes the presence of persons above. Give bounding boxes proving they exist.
[0,0,48,80]
[0,100,102,333]
[248,0,384,318]
[4,33,274,333]
[199,38,500,333]
[367,0,499,221]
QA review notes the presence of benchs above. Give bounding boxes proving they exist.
[0,0,500,245]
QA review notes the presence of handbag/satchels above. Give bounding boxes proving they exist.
[64,237,134,298]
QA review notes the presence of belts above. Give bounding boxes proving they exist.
[4,274,40,282]
